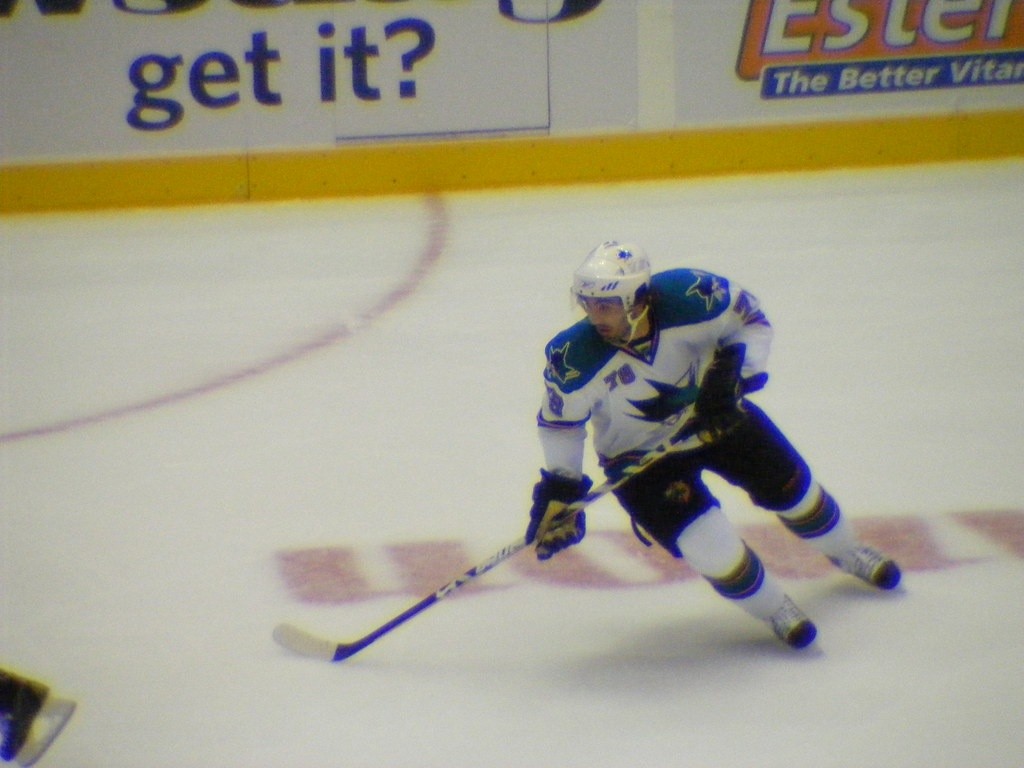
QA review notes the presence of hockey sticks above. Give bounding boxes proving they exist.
[269,368,771,665]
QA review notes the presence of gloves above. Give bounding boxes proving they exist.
[696,366,749,446]
[525,467,595,562]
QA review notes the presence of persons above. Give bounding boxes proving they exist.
[526,239,902,652]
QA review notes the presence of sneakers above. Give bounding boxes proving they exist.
[0,671,75,768]
[767,594,816,650]
[825,542,902,591]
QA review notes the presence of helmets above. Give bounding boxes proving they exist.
[572,240,650,313]
